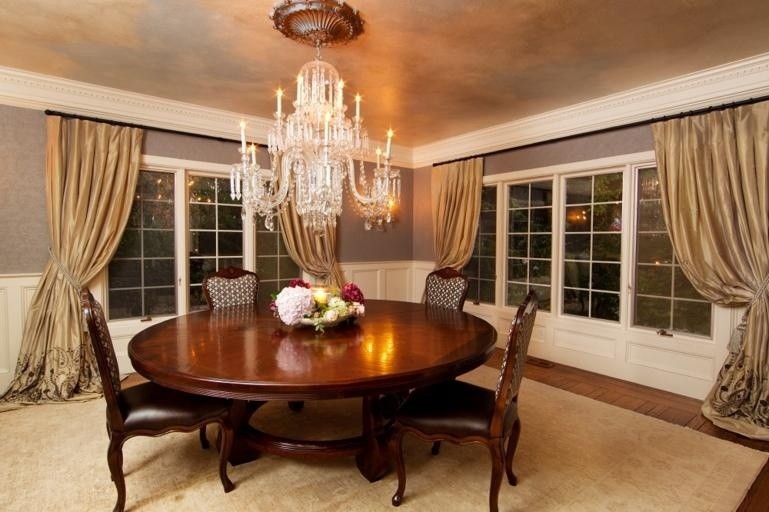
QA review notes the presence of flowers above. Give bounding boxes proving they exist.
[267,273,370,335]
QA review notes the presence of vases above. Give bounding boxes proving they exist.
[297,317,346,329]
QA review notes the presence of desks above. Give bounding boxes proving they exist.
[126,292,500,485]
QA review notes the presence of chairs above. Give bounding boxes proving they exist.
[422,266,470,311]
[391,288,541,512]
[78,285,241,512]
[200,264,262,309]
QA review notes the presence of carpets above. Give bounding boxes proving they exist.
[1,364,769,512]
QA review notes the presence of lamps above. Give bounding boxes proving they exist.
[228,0,405,240]
